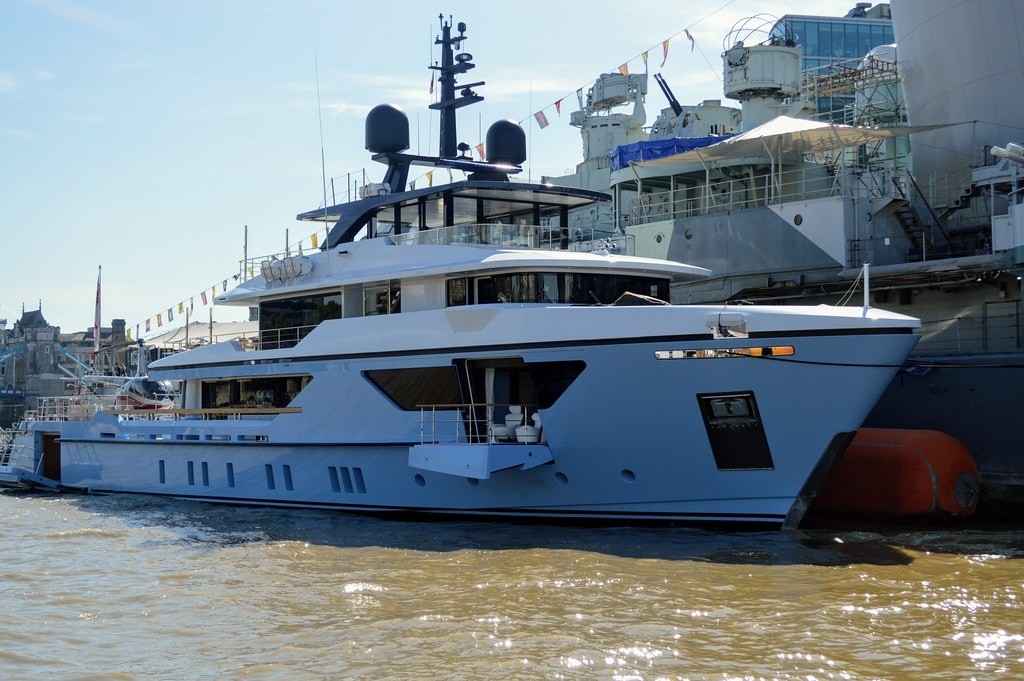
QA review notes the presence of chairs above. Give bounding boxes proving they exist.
[493,405,541,442]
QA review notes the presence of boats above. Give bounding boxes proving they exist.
[52,12,922,533]
[0,372,176,495]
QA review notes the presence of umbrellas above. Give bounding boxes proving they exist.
[697,115,977,207]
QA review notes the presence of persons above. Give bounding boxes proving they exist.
[115,362,126,377]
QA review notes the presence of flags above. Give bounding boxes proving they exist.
[93,270,101,352]
